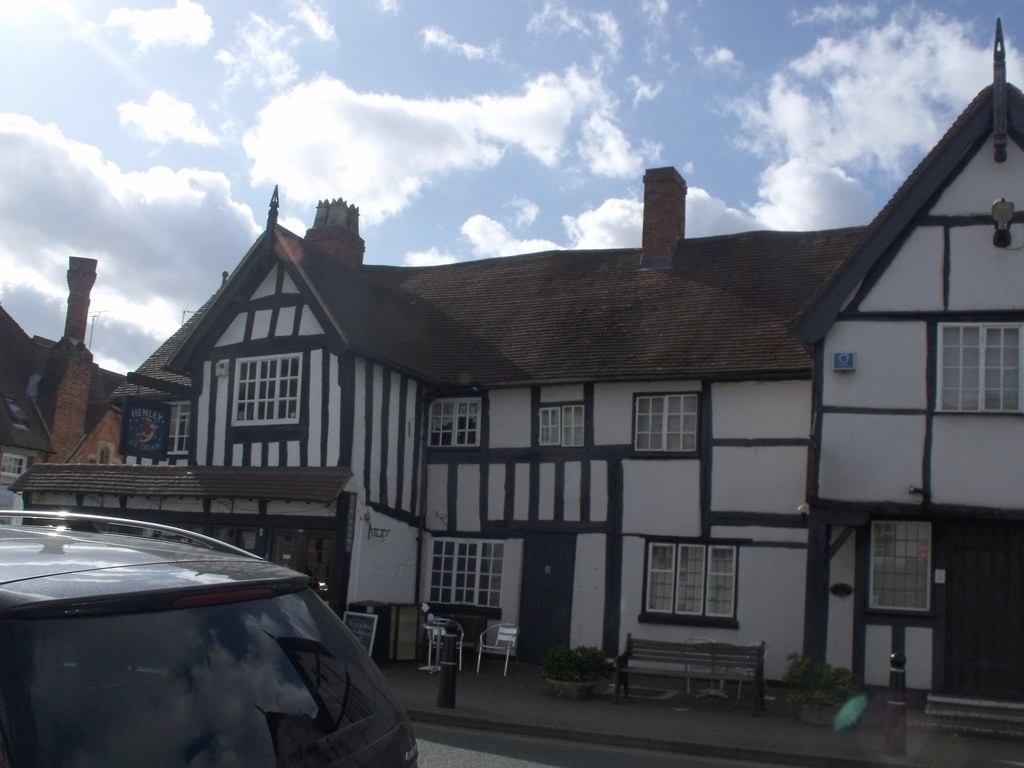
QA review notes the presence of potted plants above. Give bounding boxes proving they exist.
[537,644,617,702]
[782,651,867,728]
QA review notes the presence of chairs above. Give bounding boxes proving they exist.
[418,616,465,671]
[475,623,521,676]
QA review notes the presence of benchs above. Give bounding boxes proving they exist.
[611,632,768,719]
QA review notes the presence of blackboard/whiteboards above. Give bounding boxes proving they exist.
[342,612,378,658]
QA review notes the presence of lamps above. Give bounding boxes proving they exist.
[362,505,391,540]
[797,504,820,529]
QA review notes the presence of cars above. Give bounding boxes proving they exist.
[1,506,421,768]
